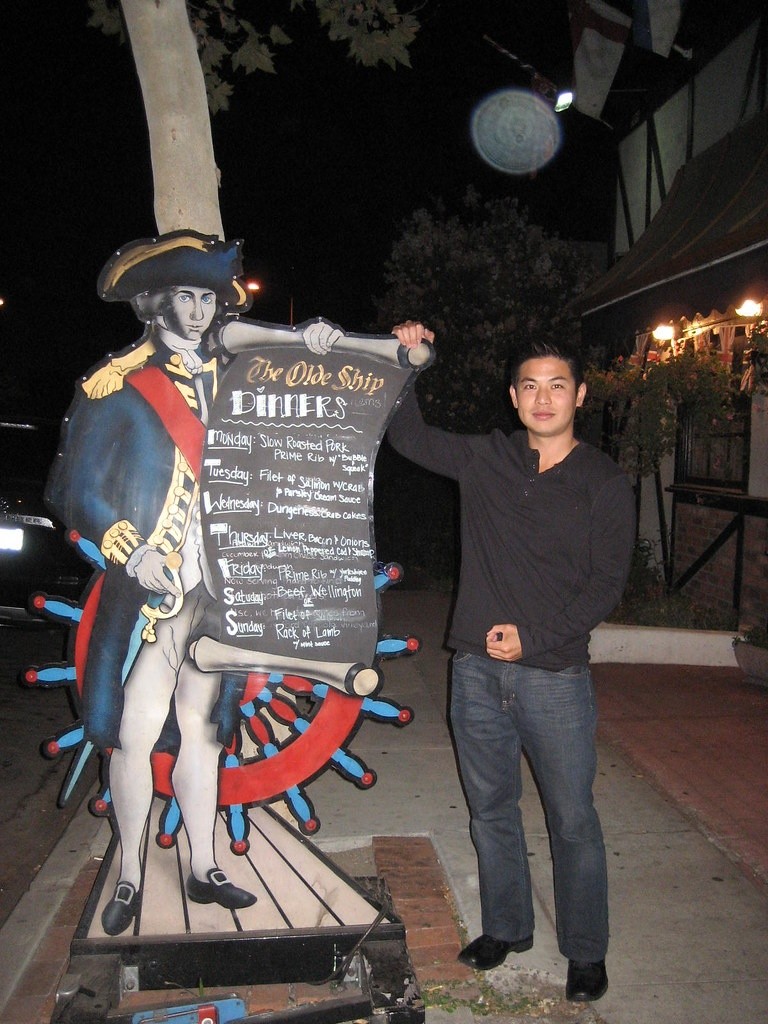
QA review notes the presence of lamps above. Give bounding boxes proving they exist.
[652,323,676,340]
[735,299,764,318]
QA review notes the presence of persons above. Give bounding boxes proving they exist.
[384,320,638,999]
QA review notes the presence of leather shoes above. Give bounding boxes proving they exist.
[566,958,608,1001]
[458,933,533,970]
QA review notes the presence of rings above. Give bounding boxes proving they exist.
[405,320,413,326]
[415,321,420,325]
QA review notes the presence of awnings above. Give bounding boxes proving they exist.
[575,112,768,329]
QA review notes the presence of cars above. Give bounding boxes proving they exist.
[0,422,91,613]
